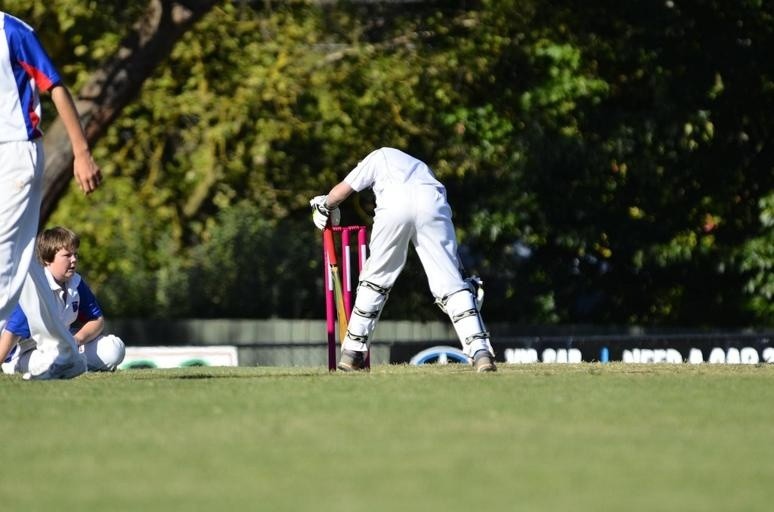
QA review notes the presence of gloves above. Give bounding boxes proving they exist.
[313,199,330,231]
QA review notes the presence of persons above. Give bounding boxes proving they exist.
[0,226,125,373]
[0,11,103,381]
[309,145,498,373]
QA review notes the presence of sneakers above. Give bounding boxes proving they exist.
[477,357,497,373]
[31,360,86,381]
[338,350,366,372]
